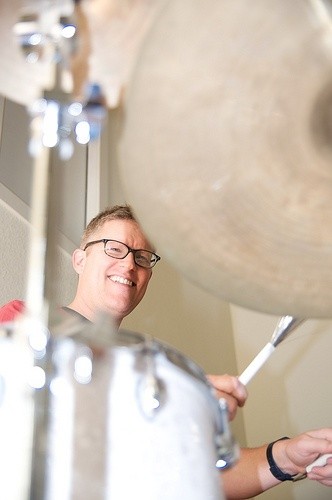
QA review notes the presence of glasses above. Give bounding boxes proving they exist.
[84,239,162,270]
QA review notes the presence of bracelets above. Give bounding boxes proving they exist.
[267,437,308,482]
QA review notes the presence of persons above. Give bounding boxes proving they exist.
[0,201,332,500]
